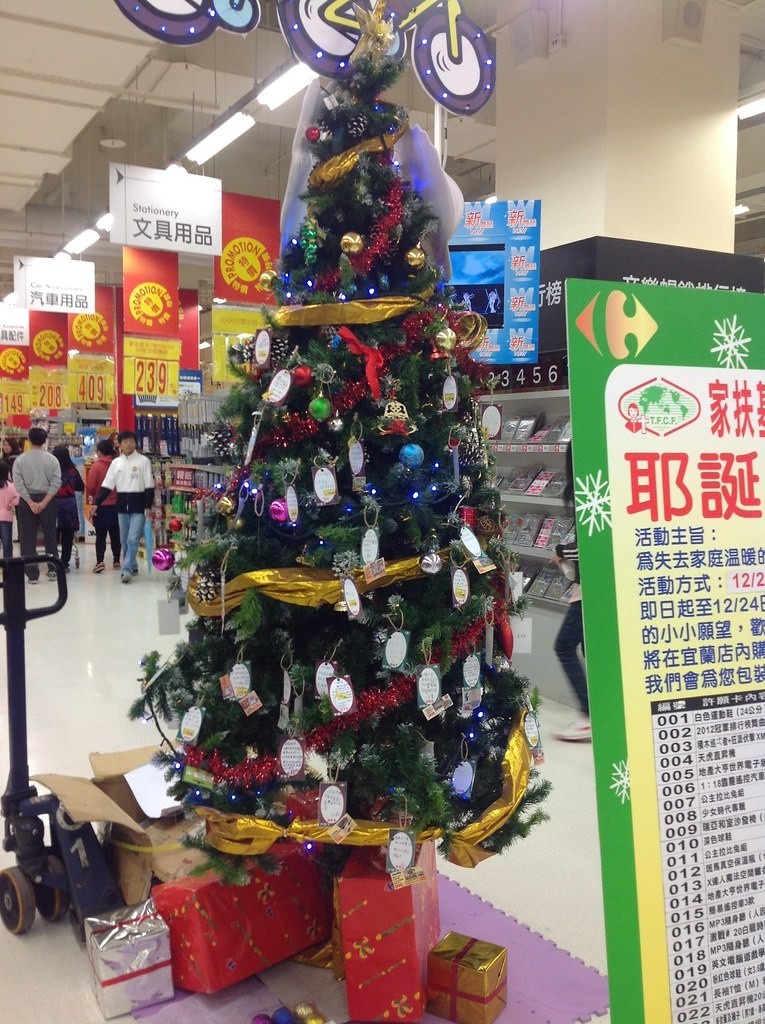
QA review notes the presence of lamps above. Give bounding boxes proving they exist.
[186,62,320,165]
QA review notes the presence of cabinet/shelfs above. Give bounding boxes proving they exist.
[168,465,225,550]
[474,388,588,712]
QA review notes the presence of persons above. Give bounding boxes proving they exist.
[0,428,157,584]
[549,439,592,741]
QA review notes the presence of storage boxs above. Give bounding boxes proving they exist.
[26,742,508,1023]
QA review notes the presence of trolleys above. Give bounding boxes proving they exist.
[52,519,80,569]
[0,556,128,949]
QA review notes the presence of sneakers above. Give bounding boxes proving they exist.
[93,562,105,572]
[551,716,592,740]
[120,568,139,583]
[28,576,38,583]
[113,559,122,570]
[47,570,57,581]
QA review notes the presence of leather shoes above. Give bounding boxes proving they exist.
[63,565,70,574]
